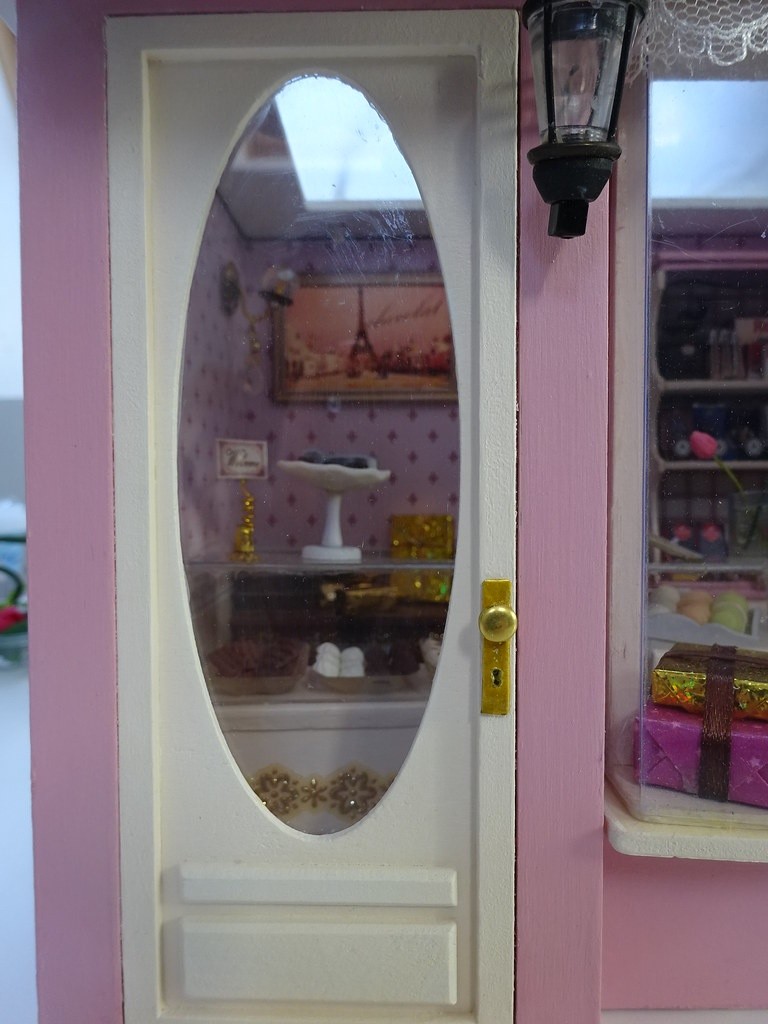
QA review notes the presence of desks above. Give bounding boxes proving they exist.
[188,550,453,647]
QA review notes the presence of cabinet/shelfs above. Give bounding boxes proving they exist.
[653,262,768,573]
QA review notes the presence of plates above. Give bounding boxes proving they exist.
[207,665,303,695]
[312,662,427,694]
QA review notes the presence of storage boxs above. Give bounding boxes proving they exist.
[631,643,768,808]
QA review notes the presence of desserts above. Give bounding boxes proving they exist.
[204,632,443,680]
[649,585,749,634]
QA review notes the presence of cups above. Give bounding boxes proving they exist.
[730,491,768,565]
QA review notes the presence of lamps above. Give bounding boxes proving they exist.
[521,0,649,239]
[222,261,298,357]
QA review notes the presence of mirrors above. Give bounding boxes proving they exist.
[173,74,464,839]
[268,270,461,404]
